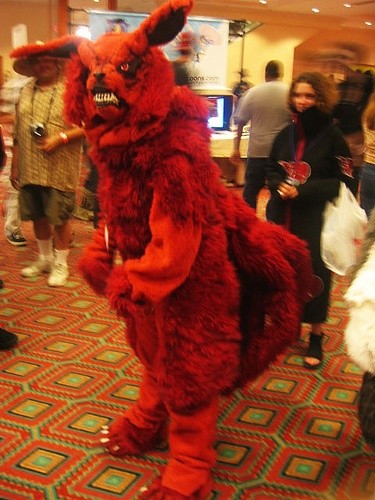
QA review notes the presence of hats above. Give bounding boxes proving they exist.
[12,41,68,78]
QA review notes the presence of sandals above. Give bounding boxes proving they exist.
[304,333,325,369]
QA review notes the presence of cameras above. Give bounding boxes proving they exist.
[31,123,45,138]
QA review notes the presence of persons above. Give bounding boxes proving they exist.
[170,47,193,90]
[8,51,87,288]
[230,59,299,227]
[3,159,76,249]
[359,87,375,223]
[265,71,357,370]
[233,68,256,125]
[326,66,374,135]
[0,326,18,349]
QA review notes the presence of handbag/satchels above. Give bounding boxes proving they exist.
[320,182,368,277]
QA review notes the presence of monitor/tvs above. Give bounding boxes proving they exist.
[198,94,233,132]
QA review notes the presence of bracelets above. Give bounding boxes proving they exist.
[59,131,69,145]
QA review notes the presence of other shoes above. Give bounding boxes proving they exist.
[0,326,18,349]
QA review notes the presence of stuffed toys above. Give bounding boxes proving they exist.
[8,0,314,500]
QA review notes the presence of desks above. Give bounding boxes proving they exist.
[210,131,251,186]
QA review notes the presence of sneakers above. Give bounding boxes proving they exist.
[22,257,51,277]
[6,232,27,246]
[48,263,69,286]
